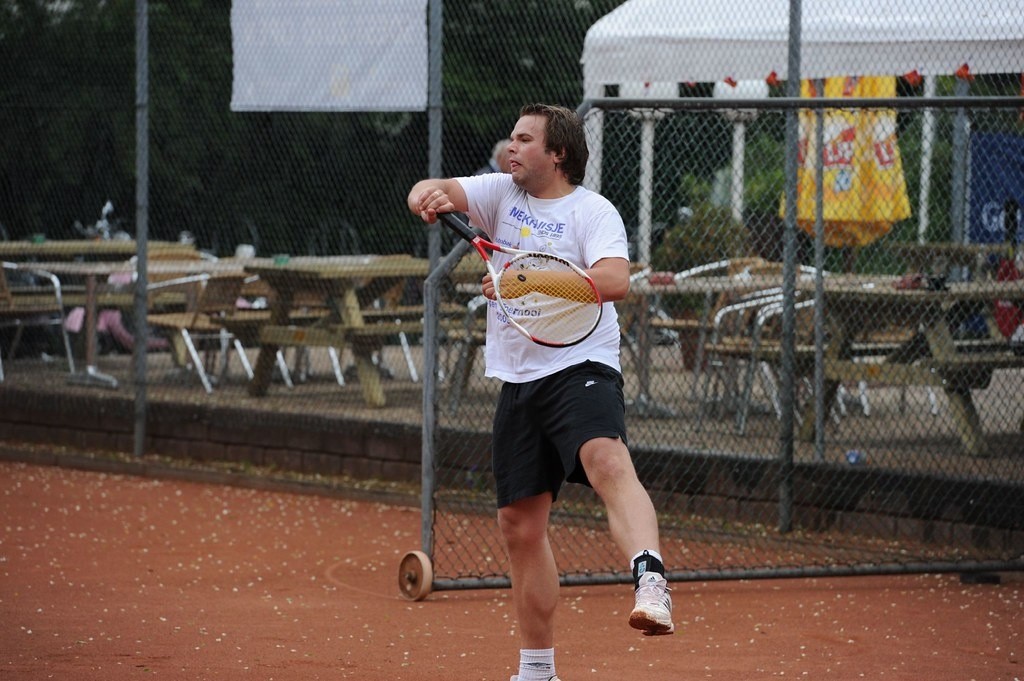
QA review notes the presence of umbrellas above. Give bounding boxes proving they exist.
[777,74,912,272]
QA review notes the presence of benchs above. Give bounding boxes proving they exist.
[1,240,1024,458]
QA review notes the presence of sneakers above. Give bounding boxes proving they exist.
[629,572,675,636]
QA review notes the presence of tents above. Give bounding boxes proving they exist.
[580,0,1024,416]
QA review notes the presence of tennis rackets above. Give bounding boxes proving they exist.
[439,204,602,347]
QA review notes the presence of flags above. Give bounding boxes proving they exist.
[229,1,430,113]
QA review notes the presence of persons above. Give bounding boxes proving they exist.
[407,100,676,681]
[62,271,171,360]
